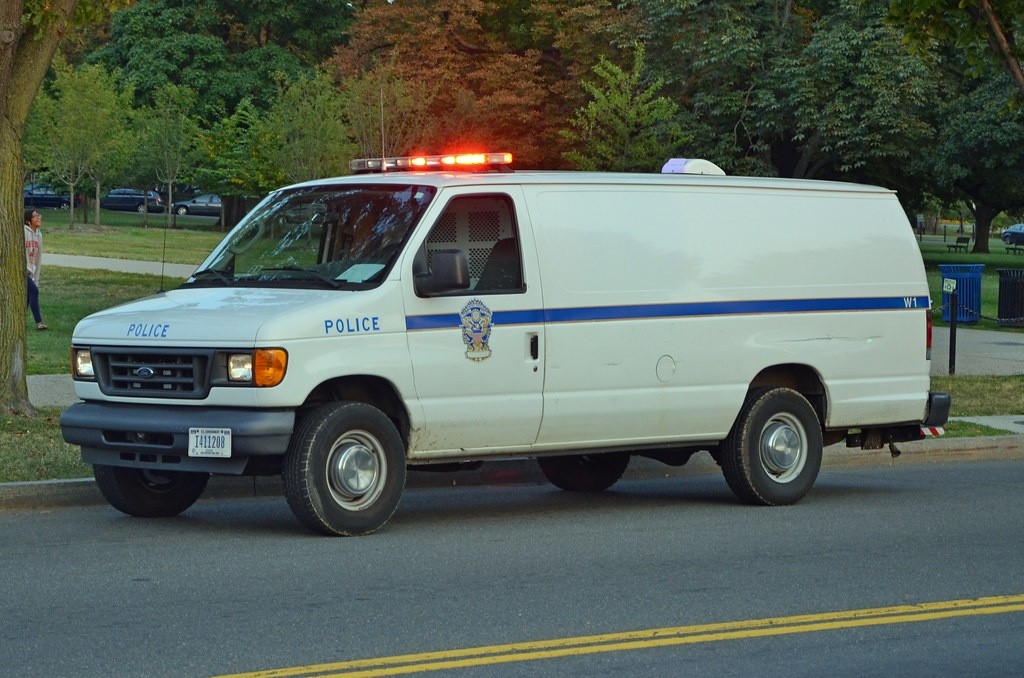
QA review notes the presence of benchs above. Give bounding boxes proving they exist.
[947,237,970,253]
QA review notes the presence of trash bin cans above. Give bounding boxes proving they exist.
[994,268,1024,328]
[936,264,987,325]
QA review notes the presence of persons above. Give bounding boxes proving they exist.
[24,210,47,328]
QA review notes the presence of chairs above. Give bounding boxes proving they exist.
[474,238,522,290]
[380,242,428,275]
[1006,239,1024,254]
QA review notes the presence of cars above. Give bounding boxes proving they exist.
[1001,224,1024,245]
[276,203,328,223]
[167,191,221,215]
[24,181,78,211]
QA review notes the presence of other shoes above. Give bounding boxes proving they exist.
[36,322,47,329]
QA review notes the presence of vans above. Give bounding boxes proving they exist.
[60,151,952,539]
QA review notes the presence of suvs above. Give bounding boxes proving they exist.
[99,187,163,214]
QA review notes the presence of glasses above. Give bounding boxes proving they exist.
[31,213,40,218]
